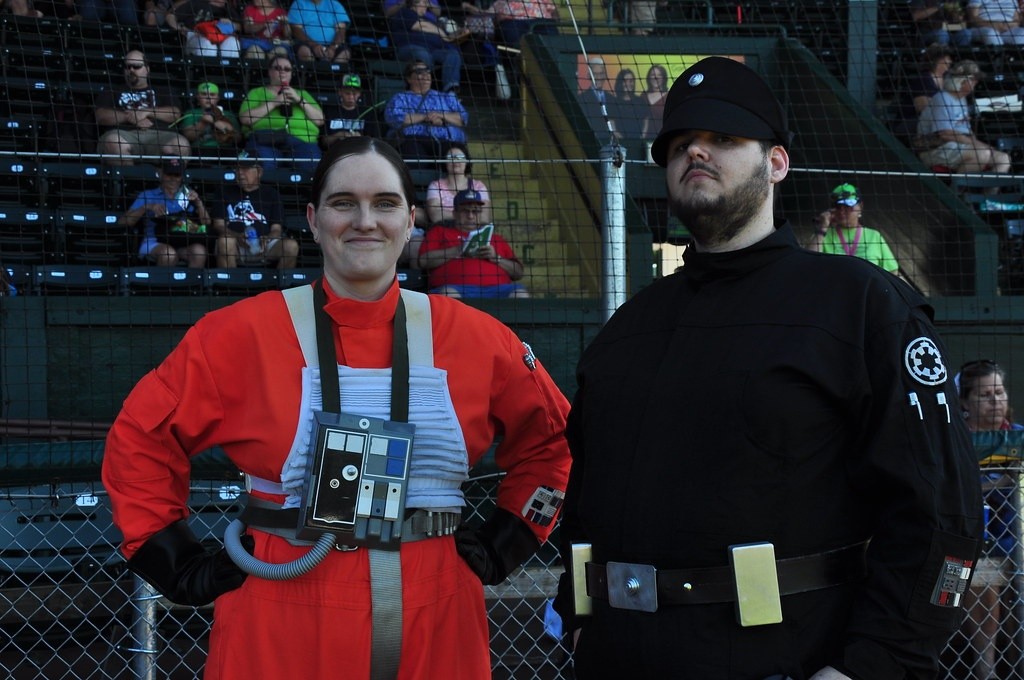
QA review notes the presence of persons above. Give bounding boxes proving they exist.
[957,358,1024,680]
[0,0,563,299]
[629,0,669,36]
[100,136,575,680]
[552,57,983,680]
[576,58,670,183]
[909,0,1024,195]
[804,182,898,277]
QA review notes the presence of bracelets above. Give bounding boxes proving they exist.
[297,97,303,104]
[815,228,826,236]
[331,42,339,49]
[491,254,501,263]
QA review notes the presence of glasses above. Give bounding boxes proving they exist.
[231,164,261,173]
[454,208,480,217]
[832,192,856,200]
[122,63,146,71]
[413,66,431,75]
[272,64,292,73]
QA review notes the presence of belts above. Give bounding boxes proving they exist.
[585,536,871,604]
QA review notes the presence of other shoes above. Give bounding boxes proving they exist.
[444,85,461,95]
[495,63,510,99]
[979,201,1009,211]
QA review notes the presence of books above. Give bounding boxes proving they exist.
[461,224,494,258]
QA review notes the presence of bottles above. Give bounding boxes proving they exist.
[279,81,293,116]
[244,221,261,254]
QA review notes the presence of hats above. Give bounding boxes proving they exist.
[651,57,790,168]
[198,78,220,94]
[224,148,263,171]
[945,60,988,80]
[342,75,363,91]
[159,153,185,177]
[455,190,485,208]
[827,182,860,208]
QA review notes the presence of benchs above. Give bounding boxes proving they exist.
[603,0,1024,244]
[0,2,447,292]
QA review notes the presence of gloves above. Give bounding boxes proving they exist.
[455,505,541,585]
[126,518,254,607]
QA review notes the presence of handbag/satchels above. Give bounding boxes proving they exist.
[149,199,216,247]
[387,131,404,148]
